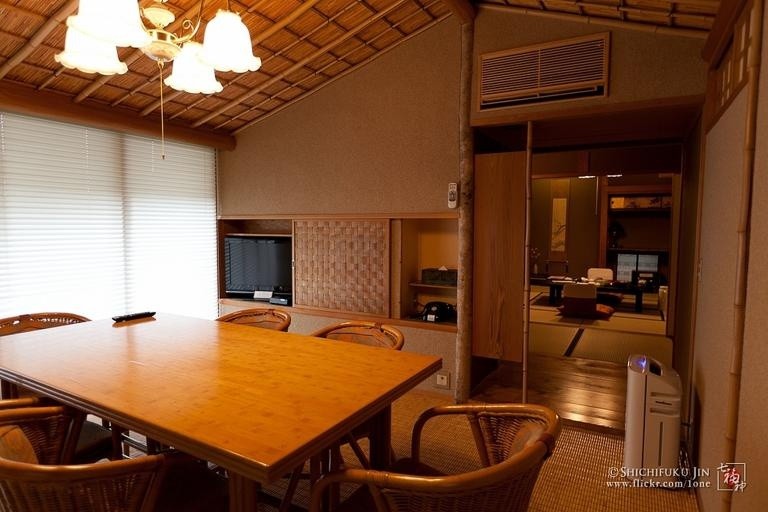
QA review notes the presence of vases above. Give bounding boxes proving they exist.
[534,263,538,274]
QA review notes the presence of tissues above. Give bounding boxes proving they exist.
[421,264,457,285]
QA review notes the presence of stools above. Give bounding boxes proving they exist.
[529,275,547,286]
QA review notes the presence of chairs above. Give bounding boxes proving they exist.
[565,283,597,318]
[588,268,613,281]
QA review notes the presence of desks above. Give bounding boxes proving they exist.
[545,278,644,312]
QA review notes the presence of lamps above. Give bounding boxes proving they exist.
[54,0,263,160]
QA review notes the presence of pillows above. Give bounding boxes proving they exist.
[558,303,616,318]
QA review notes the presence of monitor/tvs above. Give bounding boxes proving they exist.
[225,233,292,301]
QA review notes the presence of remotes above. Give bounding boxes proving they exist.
[112,311,156,323]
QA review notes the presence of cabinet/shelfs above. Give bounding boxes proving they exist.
[390,212,458,333]
[598,184,673,285]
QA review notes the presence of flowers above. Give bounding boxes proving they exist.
[530,247,540,263]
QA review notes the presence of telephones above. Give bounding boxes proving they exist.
[419,301,453,322]
